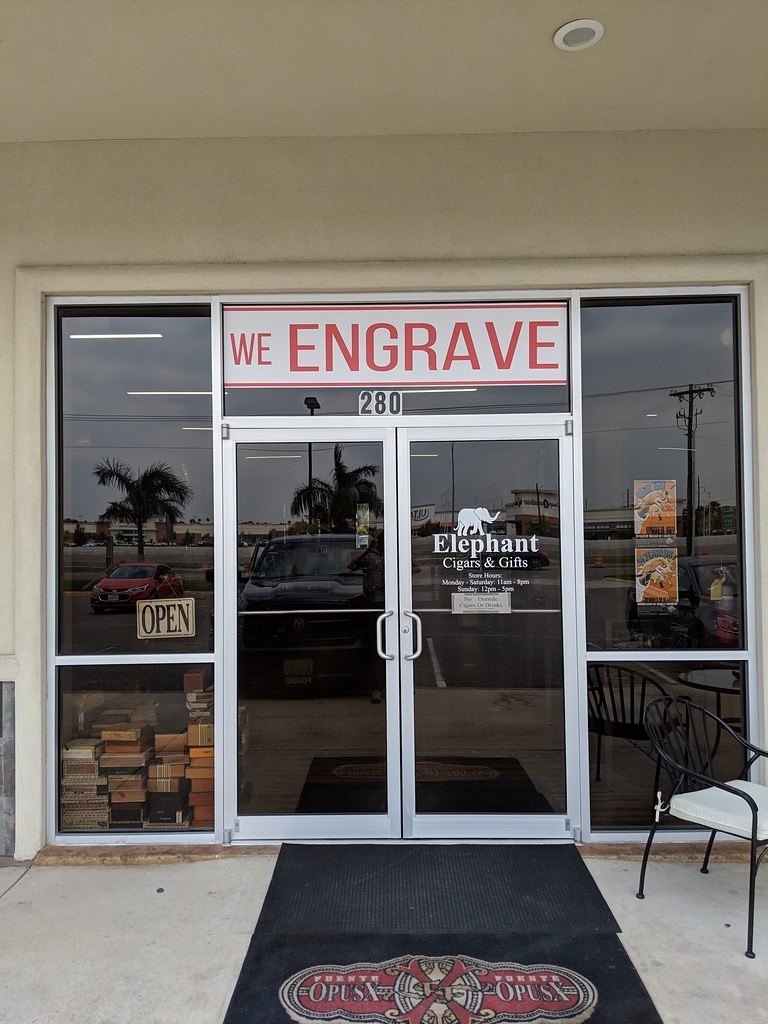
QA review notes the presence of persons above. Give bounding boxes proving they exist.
[346,528,421,705]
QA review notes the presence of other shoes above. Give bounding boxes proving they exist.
[371,689,381,703]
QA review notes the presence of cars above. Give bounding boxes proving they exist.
[89,562,184,615]
[625,554,740,649]
[206,535,374,689]
[240,538,271,547]
[491,530,506,535]
[468,544,551,570]
[64,539,178,547]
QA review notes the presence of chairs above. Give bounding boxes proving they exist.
[587,664,682,815]
[636,696,768,958]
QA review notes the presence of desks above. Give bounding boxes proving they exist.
[677,669,741,783]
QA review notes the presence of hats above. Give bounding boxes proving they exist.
[374,528,383,538]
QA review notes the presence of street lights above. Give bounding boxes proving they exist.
[304,396,321,525]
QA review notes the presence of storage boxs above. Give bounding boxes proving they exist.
[60,669,214,831]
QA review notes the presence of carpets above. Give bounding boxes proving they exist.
[221,843,662,1024]
[294,756,554,813]
